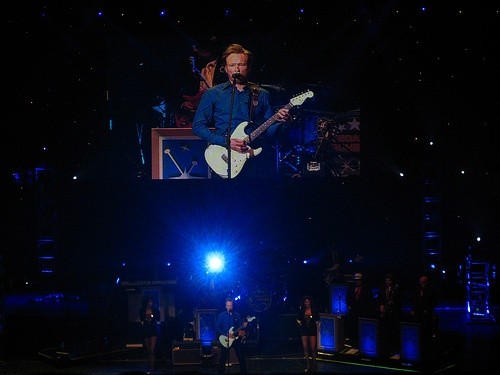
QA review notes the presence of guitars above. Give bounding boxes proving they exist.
[202,87,316,181]
[219,315,257,349]
[174,89,215,127]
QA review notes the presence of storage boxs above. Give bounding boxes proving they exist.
[172,347,203,365]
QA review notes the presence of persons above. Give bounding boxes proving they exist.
[296,297,320,373]
[344,271,434,357]
[216,298,247,375]
[139,294,160,375]
[192,43,293,180]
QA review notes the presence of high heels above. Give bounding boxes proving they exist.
[304,363,316,373]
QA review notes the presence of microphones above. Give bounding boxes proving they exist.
[190,56,195,73]
[232,72,240,78]
